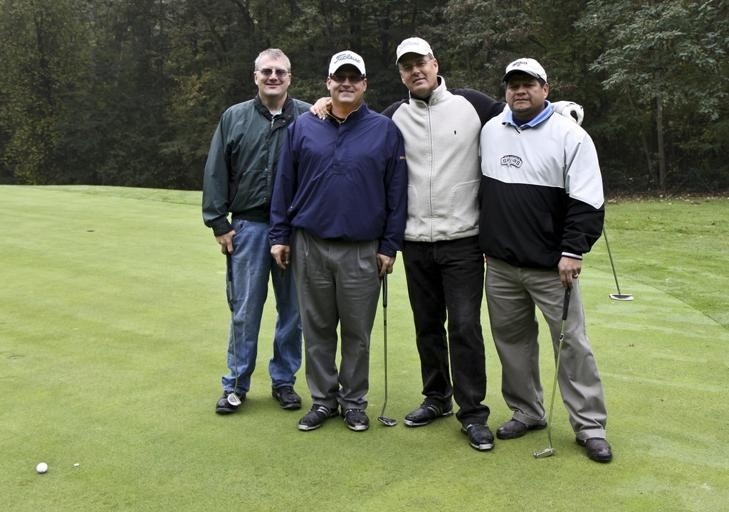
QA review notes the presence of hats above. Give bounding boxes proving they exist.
[501,56,548,86]
[327,49,367,80]
[395,35,435,66]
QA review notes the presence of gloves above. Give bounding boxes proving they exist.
[549,100,585,126]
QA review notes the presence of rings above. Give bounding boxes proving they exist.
[572,272,579,279]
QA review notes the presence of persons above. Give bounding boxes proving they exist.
[202,48,316,415]
[269,50,408,430]
[309,36,587,452]
[474,57,612,463]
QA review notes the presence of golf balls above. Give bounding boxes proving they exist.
[36,463,48,473]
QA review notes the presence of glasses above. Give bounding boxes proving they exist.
[330,73,366,82]
[253,68,290,77]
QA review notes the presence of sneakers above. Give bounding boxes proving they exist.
[270,387,302,410]
[341,406,370,432]
[403,396,454,430]
[497,418,550,440]
[215,389,246,415]
[298,403,339,432]
[576,437,612,464]
[461,421,494,452]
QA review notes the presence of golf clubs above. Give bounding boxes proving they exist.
[534,286,572,460]
[570,110,634,300]
[378,273,398,427]
[226,251,242,407]
